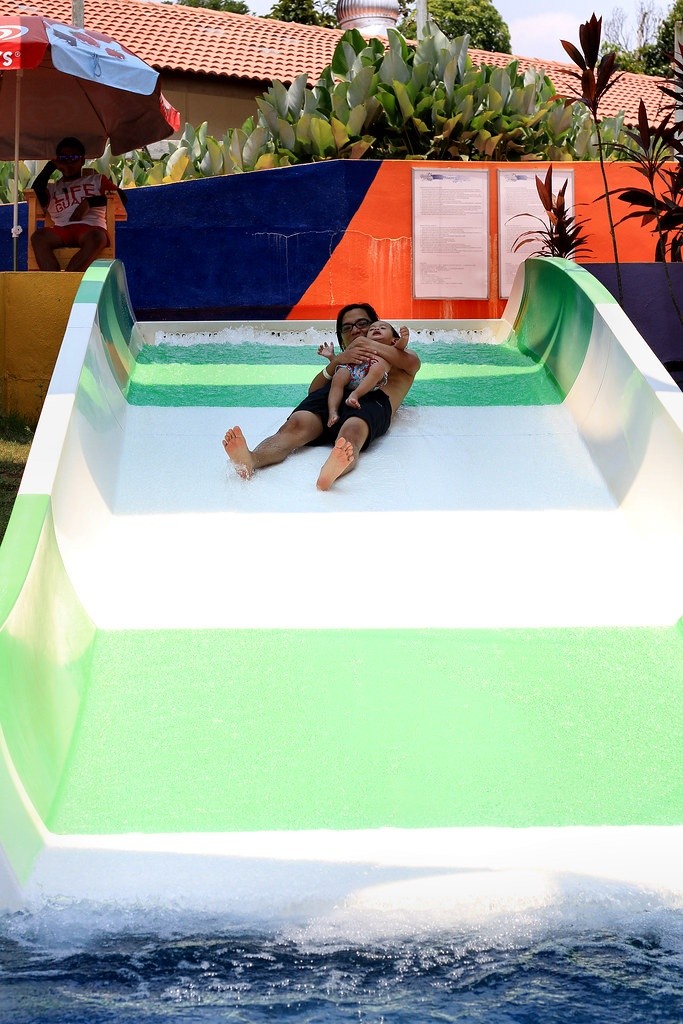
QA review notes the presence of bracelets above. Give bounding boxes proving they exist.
[323,367,332,380]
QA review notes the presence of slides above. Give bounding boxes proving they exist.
[0,257,683,938]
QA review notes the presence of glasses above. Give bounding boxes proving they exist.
[57,155,84,163]
[340,318,372,334]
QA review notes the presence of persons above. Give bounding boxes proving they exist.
[30,137,129,272]
[221,303,421,490]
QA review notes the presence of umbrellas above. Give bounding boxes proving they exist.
[0,15,180,272]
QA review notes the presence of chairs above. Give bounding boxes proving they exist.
[21,181,128,272]
